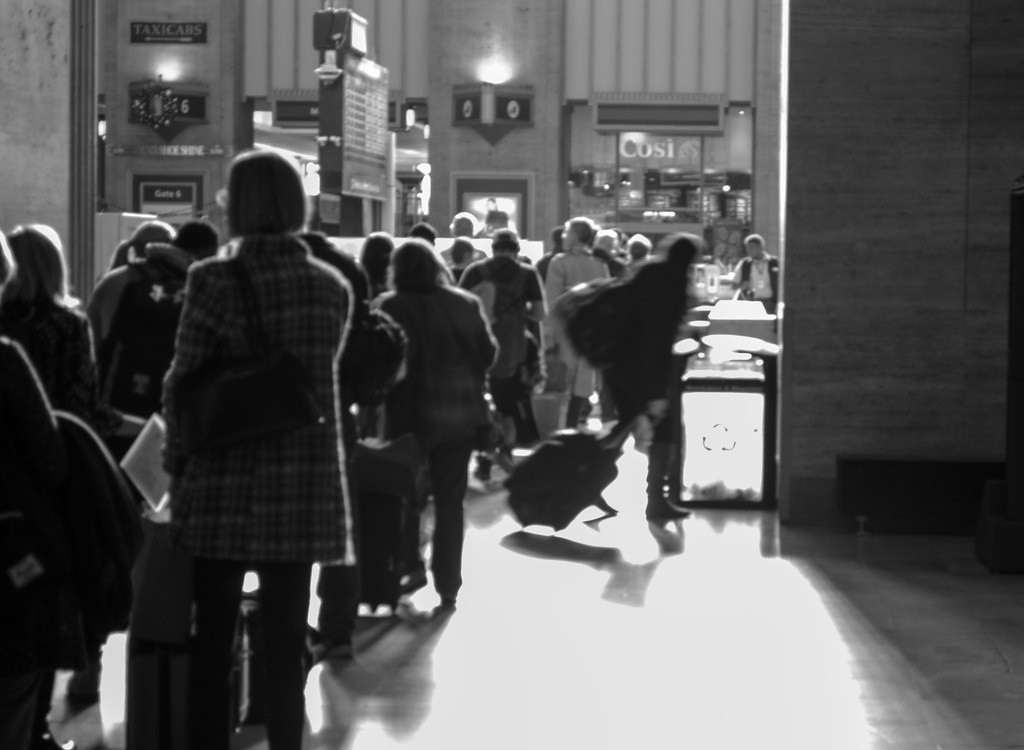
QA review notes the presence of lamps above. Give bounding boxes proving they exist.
[313,47,346,82]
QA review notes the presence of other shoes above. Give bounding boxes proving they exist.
[473,455,492,480]
[496,444,516,470]
[592,493,619,516]
[402,569,427,594]
[646,496,691,518]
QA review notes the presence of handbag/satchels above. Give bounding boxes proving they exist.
[551,275,648,368]
[177,262,324,454]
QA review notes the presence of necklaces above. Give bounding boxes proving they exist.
[755,262,766,276]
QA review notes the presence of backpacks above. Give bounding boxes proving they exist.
[470,260,527,325]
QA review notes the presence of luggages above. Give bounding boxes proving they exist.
[316,402,408,613]
[128,646,201,750]
[504,406,667,533]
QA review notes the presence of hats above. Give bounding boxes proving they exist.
[492,230,520,251]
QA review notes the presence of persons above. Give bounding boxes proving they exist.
[0,210,651,750]
[161,152,353,750]
[591,234,700,521]
[732,233,779,334]
